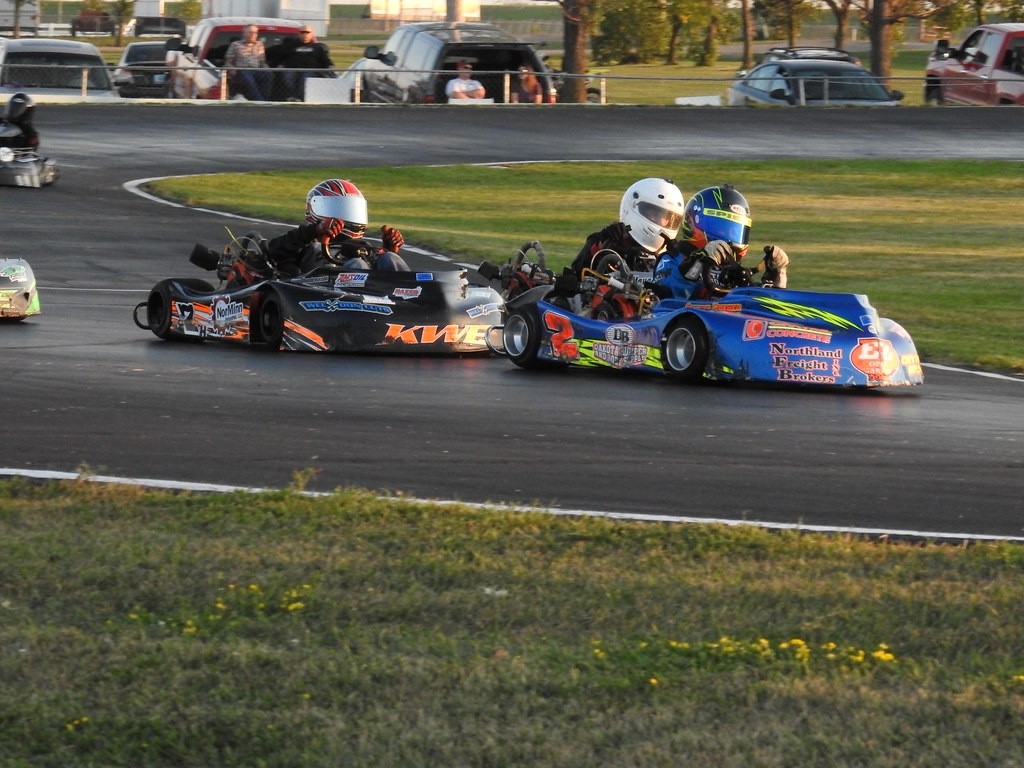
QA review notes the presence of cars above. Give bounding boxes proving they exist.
[1,38,122,100]
[726,62,904,108]
[109,41,168,99]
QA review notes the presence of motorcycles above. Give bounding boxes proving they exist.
[537,42,606,104]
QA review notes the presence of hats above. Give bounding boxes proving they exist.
[457,59,474,67]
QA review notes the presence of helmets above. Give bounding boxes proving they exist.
[306,179,368,240]
[682,185,751,259]
[7,94,33,122]
[620,178,687,253]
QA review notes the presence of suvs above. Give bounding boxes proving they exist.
[351,20,556,104]
[737,47,863,78]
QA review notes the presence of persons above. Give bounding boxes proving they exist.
[284,25,330,100]
[446,60,486,99]
[570,177,685,276]
[225,26,275,101]
[653,184,789,301]
[268,179,405,275]
[511,63,542,103]
[0,93,39,151]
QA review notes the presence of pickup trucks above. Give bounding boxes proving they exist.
[165,15,339,100]
[923,22,1024,105]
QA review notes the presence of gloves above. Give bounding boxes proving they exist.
[600,222,627,245]
[679,240,733,280]
[382,224,404,253]
[314,219,343,239]
[763,245,788,288]
